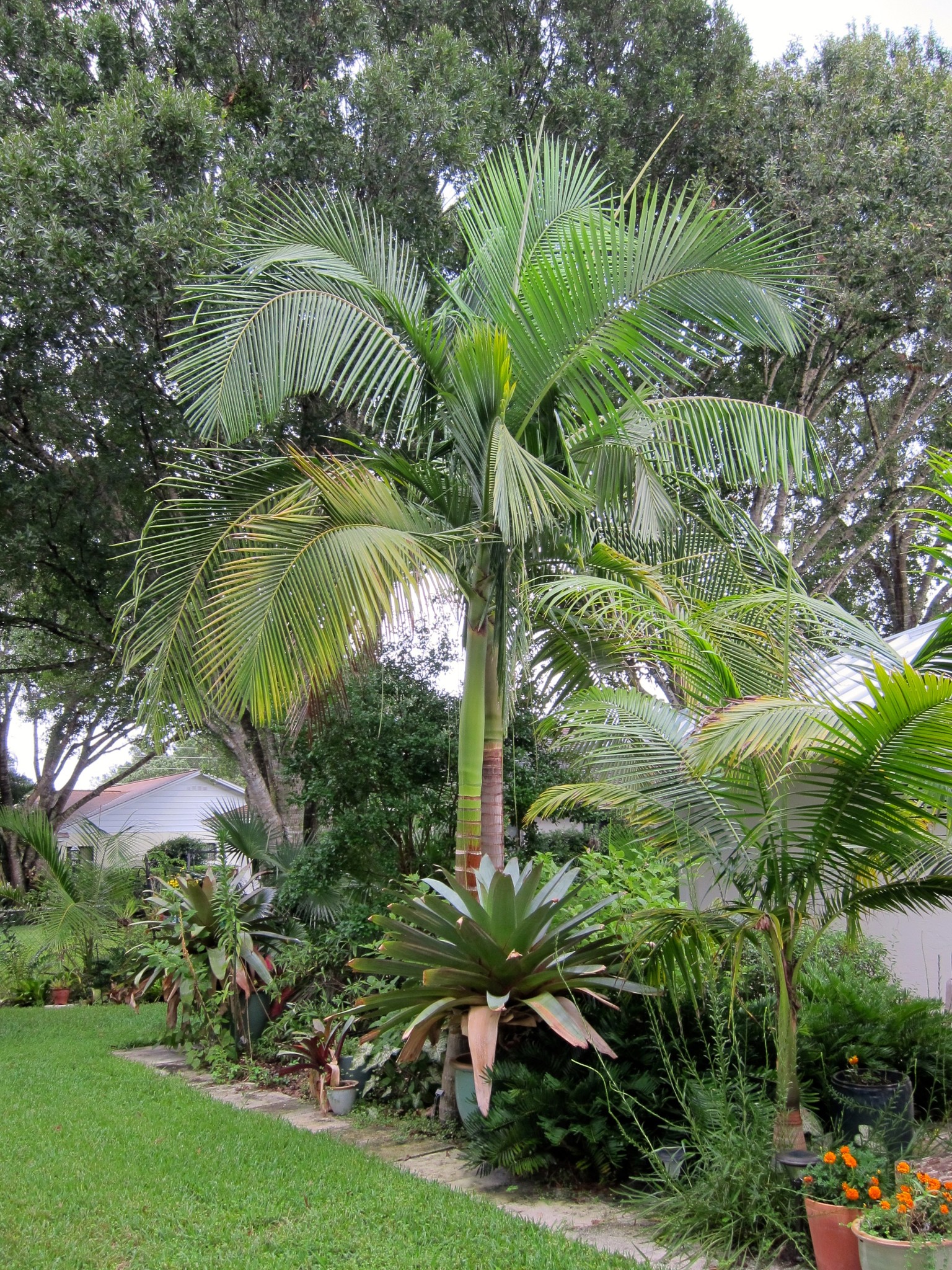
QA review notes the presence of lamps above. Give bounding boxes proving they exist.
[776,1147,820,1187]
[655,1145,684,1179]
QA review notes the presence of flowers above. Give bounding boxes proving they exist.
[794,1141,952,1270]
[844,1053,898,1083]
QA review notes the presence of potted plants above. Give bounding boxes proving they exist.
[270,1014,359,1116]
[322,852,659,1141]
[48,966,81,1005]
[109,857,303,1042]
[332,1029,374,1091]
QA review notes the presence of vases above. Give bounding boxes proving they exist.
[803,1192,872,1270]
[830,1068,914,1164]
[851,1215,952,1270]
